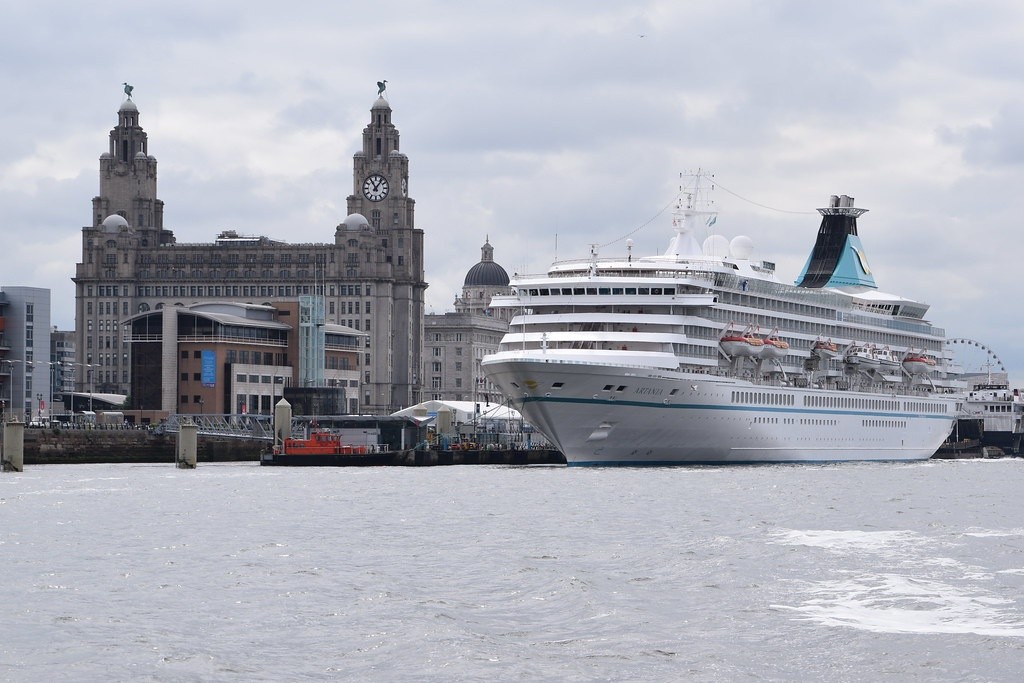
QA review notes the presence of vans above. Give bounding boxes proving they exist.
[29,415,50,428]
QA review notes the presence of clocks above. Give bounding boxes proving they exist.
[362,175,389,203]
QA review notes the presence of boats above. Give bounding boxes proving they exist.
[948,356,1024,455]
[259,411,413,467]
[846,346,881,371]
[721,335,762,357]
[813,341,838,360]
[411,377,568,464]
[874,350,902,372]
[901,356,937,373]
[756,339,790,360]
[477,167,968,462]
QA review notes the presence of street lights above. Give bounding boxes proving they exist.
[25,361,42,422]
[240,397,244,414]
[65,361,82,424]
[2,359,24,422]
[37,392,43,427]
[140,404,143,424]
[44,361,62,421]
[84,363,101,410]
[199,396,204,414]
